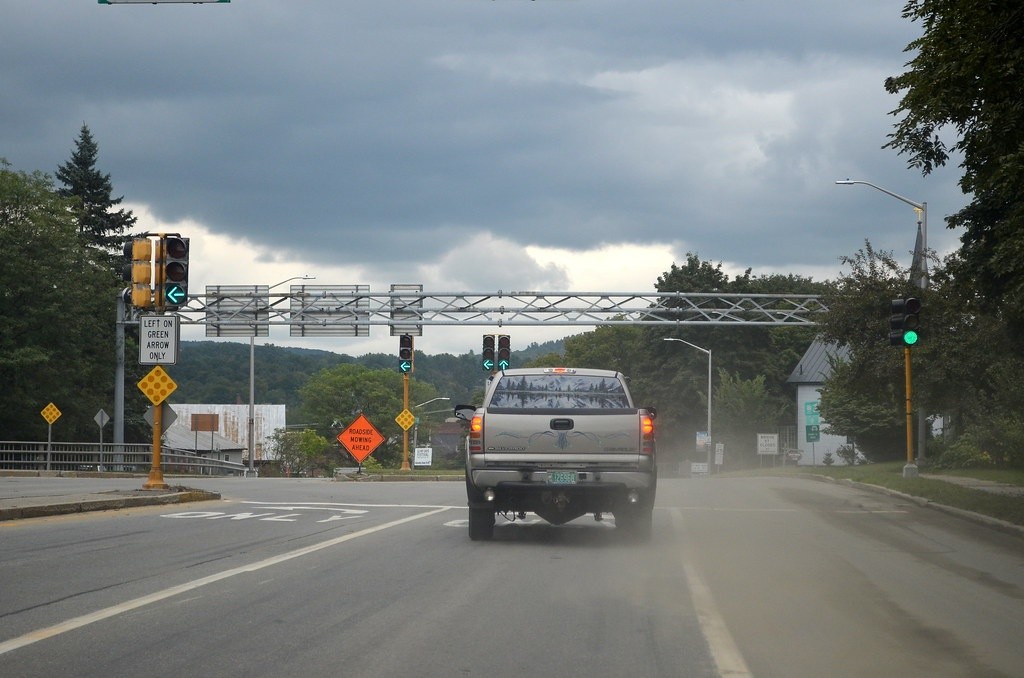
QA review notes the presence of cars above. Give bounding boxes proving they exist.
[451,363,662,544]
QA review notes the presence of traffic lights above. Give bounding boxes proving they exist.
[481,335,496,373]
[887,299,904,347]
[901,297,920,345]
[161,237,191,307]
[498,334,510,370]
[399,334,412,373]
[121,236,152,309]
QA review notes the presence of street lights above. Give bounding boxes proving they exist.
[833,179,933,291]
[245,276,316,476]
[664,336,712,474]
[413,394,450,470]
